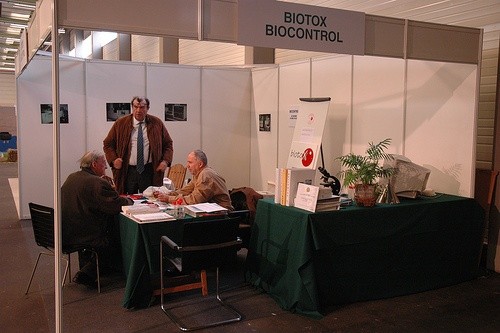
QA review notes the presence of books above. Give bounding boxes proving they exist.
[121,166,316,224]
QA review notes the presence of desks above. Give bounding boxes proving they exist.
[243,192,485,320]
[112,212,240,310]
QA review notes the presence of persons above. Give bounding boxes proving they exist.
[153,150,236,213]
[61,150,135,289]
[103,96,173,194]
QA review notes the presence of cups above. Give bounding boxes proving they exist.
[175,206,184,218]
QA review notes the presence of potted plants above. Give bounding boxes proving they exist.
[335,137,399,207]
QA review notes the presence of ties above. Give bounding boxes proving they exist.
[137,122,145,172]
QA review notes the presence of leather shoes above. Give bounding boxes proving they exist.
[72,271,98,290]
[100,271,124,278]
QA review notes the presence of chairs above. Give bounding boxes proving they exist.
[229,186,263,228]
[25,202,101,295]
[160,217,243,332]
[167,163,187,191]
